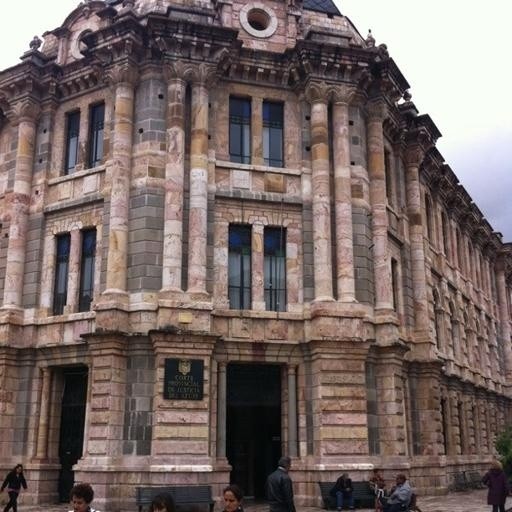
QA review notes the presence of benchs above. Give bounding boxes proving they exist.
[135,486,216,512]
[453,472,485,491]
[318,481,388,511]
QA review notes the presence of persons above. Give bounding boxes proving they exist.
[382,472,412,512]
[0,463,28,512]
[67,482,100,512]
[221,484,245,512]
[369,467,386,512]
[330,473,356,512]
[265,456,297,512]
[150,493,174,512]
[482,460,508,512]
